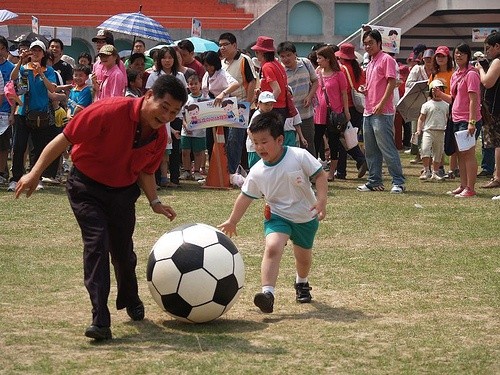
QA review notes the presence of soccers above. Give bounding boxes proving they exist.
[146,221,247,325]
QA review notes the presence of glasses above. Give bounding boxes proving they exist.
[218,42,232,47]
[100,54,111,58]
[280,53,292,59]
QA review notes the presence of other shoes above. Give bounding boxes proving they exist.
[254,291,274,312]
[0,160,206,191]
[125,299,145,321]
[294,280,312,303]
[317,144,500,198]
[84,325,112,341]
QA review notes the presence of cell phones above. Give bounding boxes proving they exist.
[26,50,33,55]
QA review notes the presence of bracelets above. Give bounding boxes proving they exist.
[149,199,161,208]
[222,91,227,96]
[41,75,45,79]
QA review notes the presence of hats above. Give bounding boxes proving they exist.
[474,51,487,57]
[9,49,19,57]
[45,50,54,61]
[29,41,46,54]
[258,91,277,104]
[429,80,447,91]
[407,44,449,63]
[334,43,358,59]
[250,36,276,52]
[60,55,75,69]
[99,45,118,55]
[92,30,113,42]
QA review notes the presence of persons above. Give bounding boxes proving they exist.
[357,29,407,194]
[403,44,460,180]
[217,111,328,313]
[65,28,128,176]
[125,40,241,188]
[0,35,75,192]
[14,74,188,342]
[472,32,500,200]
[432,42,483,198]
[279,41,368,188]
[219,32,290,184]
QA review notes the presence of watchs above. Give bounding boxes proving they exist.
[469,120,476,125]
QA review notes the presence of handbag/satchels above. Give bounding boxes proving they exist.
[339,120,359,151]
[285,91,298,118]
[342,65,366,113]
[326,107,347,131]
[443,118,458,155]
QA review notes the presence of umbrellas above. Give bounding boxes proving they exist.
[117,50,132,58]
[0,10,50,48]
[396,80,429,124]
[144,36,220,56]
[97,4,174,59]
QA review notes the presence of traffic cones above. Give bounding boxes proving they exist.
[200,126,234,190]
